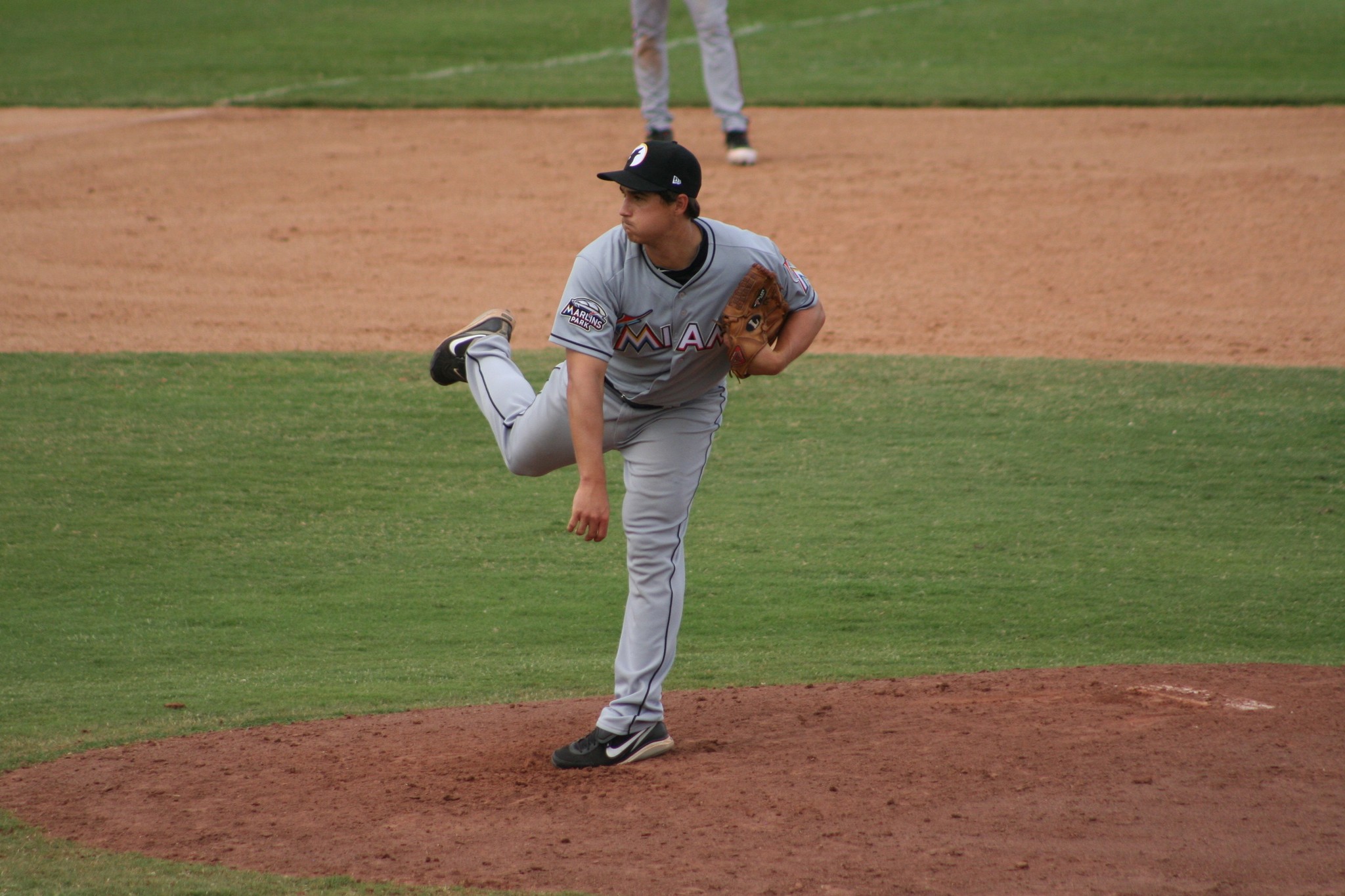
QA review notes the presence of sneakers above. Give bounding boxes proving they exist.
[552,722,676,768]
[430,309,515,386]
[726,130,758,163]
[646,132,675,143]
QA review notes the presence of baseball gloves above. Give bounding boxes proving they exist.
[720,263,789,375]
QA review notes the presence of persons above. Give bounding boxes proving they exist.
[431,139,825,769]
[629,0,758,168]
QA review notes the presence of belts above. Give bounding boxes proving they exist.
[604,376,665,409]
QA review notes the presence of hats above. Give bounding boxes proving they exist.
[597,141,701,198]
